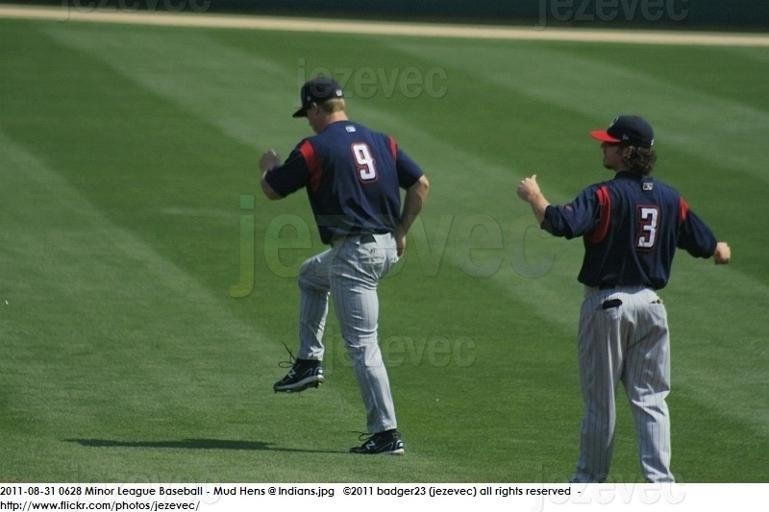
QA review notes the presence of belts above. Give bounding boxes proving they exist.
[599,283,615,290]
[358,234,376,244]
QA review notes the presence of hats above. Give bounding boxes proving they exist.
[590,115,655,148]
[292,77,345,117]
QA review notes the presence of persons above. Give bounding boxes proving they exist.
[257,75,430,456]
[517,114,731,483]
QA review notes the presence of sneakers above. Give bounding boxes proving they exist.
[272,342,325,393]
[349,430,405,455]
